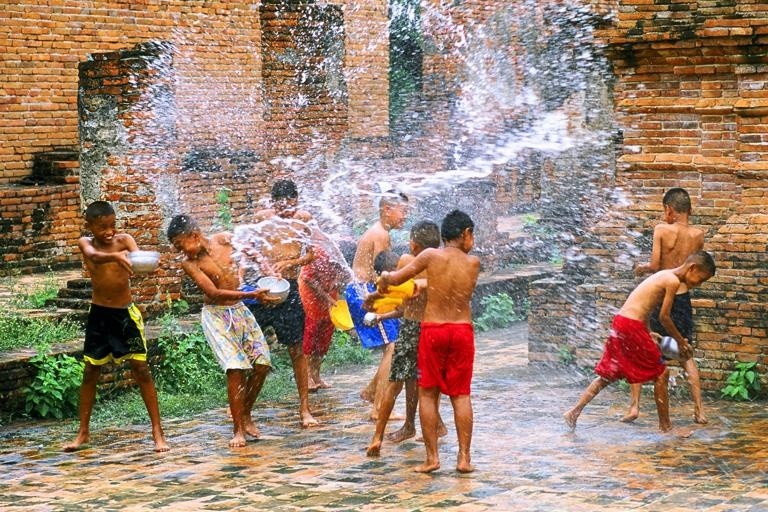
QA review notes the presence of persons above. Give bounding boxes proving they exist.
[379,210,482,476]
[167,214,284,449]
[619,188,709,426]
[298,200,350,394]
[563,249,719,440]
[367,220,446,456]
[238,179,319,427]
[342,192,408,420]
[62,200,171,452]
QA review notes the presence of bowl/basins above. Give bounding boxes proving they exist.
[371,276,416,315]
[126,250,162,274]
[329,299,355,332]
[255,275,291,303]
[660,335,682,361]
